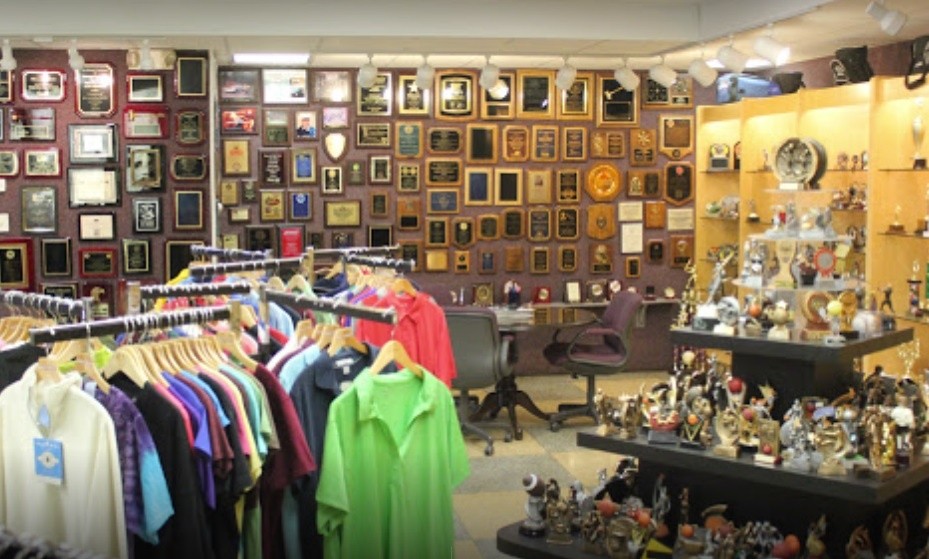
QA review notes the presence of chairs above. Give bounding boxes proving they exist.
[541,289,644,433]
[442,311,513,457]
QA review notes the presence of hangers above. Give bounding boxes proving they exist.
[0,244,425,389]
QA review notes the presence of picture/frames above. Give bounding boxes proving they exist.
[120,237,153,276]
[276,223,307,260]
[217,233,240,250]
[81,282,116,322]
[329,230,356,250]
[215,64,424,232]
[243,224,275,254]
[163,239,207,286]
[397,238,424,272]
[307,230,325,250]
[0,235,37,292]
[424,67,694,309]
[39,235,74,280]
[77,247,119,280]
[0,56,210,241]
[366,222,393,248]
[38,282,80,301]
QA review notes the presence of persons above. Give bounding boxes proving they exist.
[670,166,689,200]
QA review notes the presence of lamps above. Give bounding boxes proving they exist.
[864,0,910,38]
[687,47,718,89]
[134,38,161,75]
[414,55,436,91]
[478,54,503,92]
[613,58,641,93]
[0,39,18,71]
[716,38,750,74]
[64,42,87,72]
[750,24,790,69]
[552,56,578,93]
[355,54,380,89]
[647,55,678,88]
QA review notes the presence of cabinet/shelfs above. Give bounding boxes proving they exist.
[695,74,929,382]
[494,313,929,559]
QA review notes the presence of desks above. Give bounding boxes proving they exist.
[461,300,611,441]
